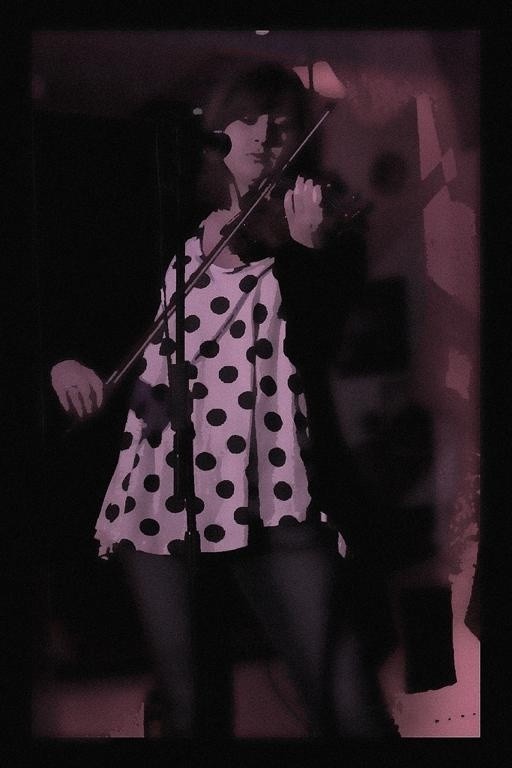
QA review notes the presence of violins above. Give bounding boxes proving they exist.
[240,165,372,251]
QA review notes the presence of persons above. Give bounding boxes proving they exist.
[48,62,404,738]
